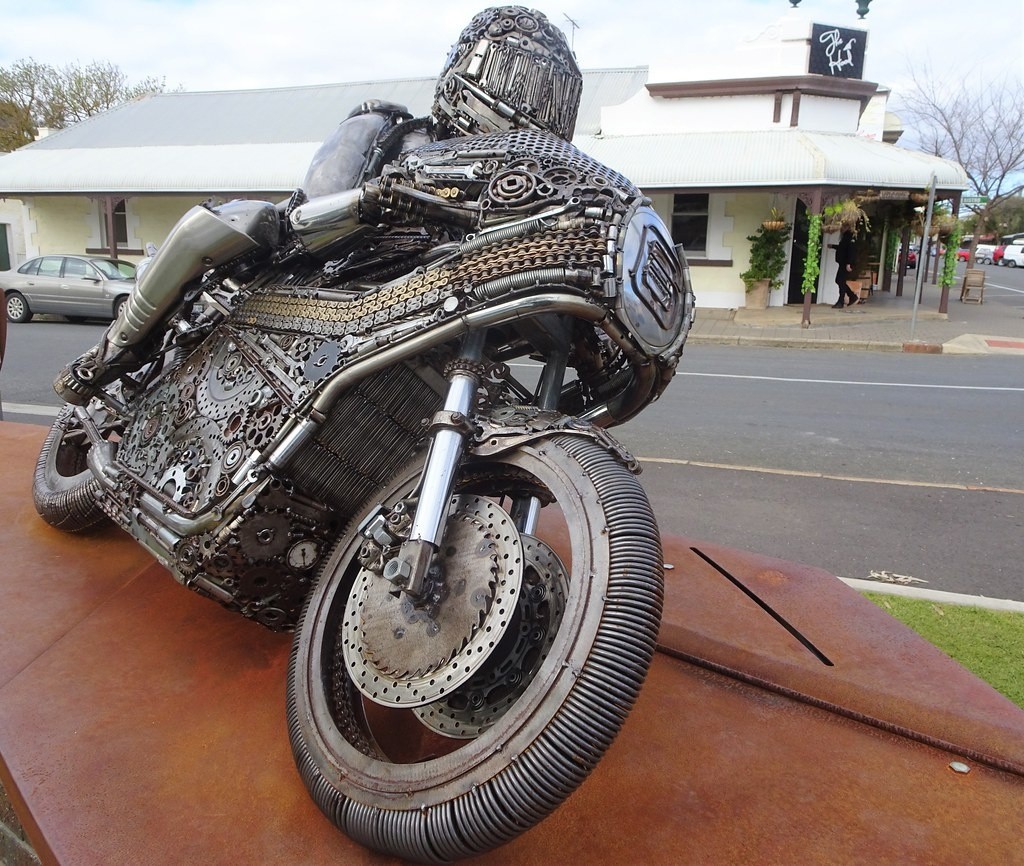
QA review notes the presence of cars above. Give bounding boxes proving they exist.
[933,238,1006,267]
[0,253,136,324]
[898,246,916,269]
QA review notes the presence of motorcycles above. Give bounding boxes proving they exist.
[37,138,694,864]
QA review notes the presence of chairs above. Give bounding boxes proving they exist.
[961,269,986,306]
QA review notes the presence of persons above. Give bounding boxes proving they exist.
[827,223,858,310]
[49,10,586,407]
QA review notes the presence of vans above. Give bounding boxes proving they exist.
[1001,239,1024,268]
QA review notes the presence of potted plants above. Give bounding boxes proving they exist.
[911,206,941,237]
[935,204,956,238]
[738,205,794,312]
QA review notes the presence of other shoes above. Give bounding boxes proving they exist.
[831,303,845,309]
[846,293,860,307]
